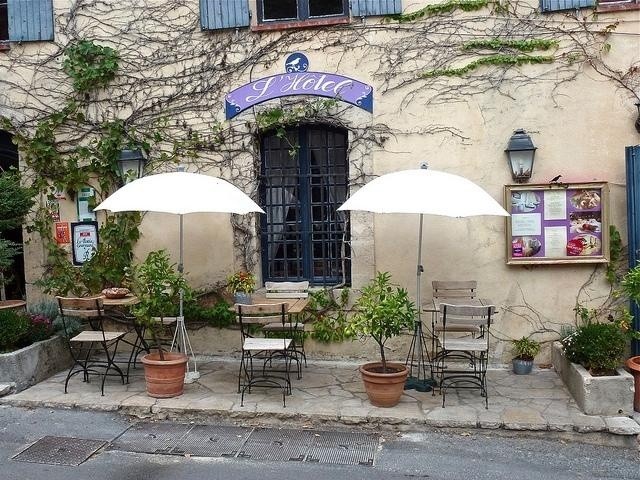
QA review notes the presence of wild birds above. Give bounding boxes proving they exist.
[549,175,563,183]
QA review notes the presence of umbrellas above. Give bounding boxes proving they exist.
[87,168,268,372]
[335,162,512,381]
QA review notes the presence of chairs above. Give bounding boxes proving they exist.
[435,302,500,409]
[134,282,182,369]
[261,280,310,369]
[432,280,482,378]
[231,301,295,407]
[55,294,124,395]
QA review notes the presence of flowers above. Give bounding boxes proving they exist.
[226,269,257,294]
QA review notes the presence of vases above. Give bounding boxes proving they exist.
[343,268,417,407]
[234,291,252,305]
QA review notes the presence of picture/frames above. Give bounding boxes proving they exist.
[502,181,611,267]
[70,221,99,266]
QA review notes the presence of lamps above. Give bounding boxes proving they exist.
[111,143,149,186]
[504,127,538,181]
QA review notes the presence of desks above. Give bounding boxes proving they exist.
[229,296,313,394]
[79,286,146,386]
[423,296,499,392]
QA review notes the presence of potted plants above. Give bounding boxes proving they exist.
[513,337,540,375]
[618,262,640,415]
[119,248,201,397]
[0,168,39,323]
